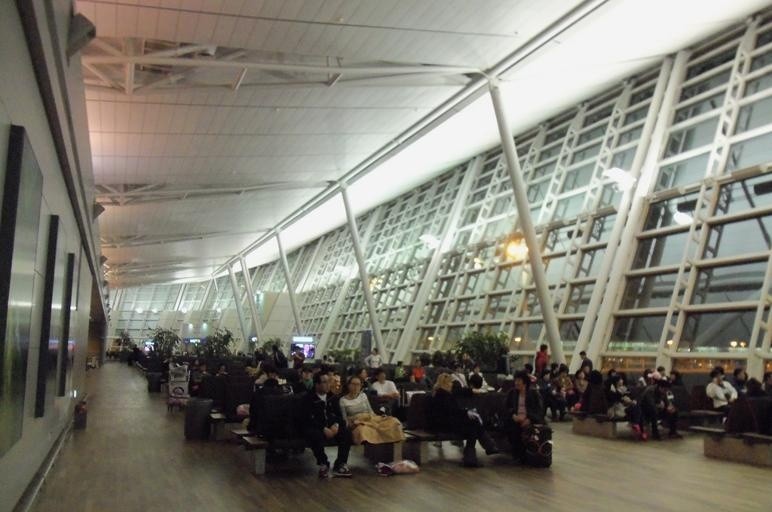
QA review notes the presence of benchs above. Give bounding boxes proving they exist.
[185,368,772,475]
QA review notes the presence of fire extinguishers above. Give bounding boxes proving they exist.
[74,401,87,430]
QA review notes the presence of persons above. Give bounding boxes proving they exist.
[190,343,555,483]
[542,347,770,443]
[133,344,139,351]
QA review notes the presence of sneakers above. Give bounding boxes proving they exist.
[332,463,353,476]
[319,462,330,480]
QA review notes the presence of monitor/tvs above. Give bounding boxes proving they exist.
[144,341,154,352]
[294,343,315,359]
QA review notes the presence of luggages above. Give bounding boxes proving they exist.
[518,421,554,469]
[168,362,192,411]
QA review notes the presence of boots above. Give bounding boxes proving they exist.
[463,446,479,466]
[480,434,499,455]
